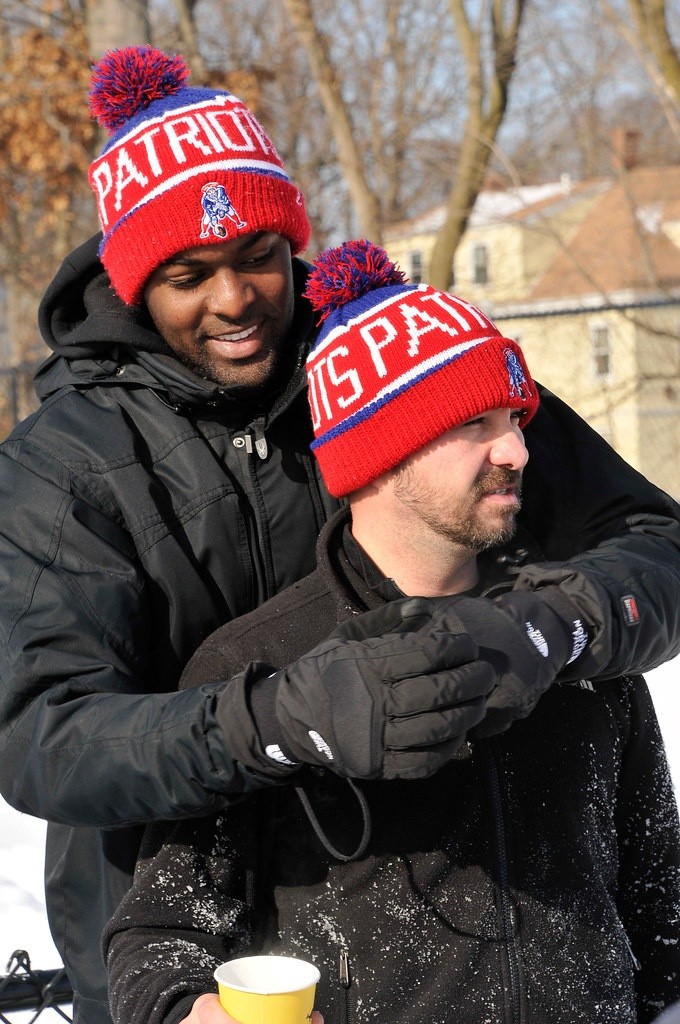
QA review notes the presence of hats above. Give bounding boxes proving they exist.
[89,47,312,305]
[305,240,540,499]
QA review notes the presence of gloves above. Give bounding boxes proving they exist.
[412,585,583,736]
[248,596,497,780]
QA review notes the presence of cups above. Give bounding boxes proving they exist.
[213,955,321,1024]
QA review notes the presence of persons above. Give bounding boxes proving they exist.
[101,241,680,1024]
[1,45,679,1023]
